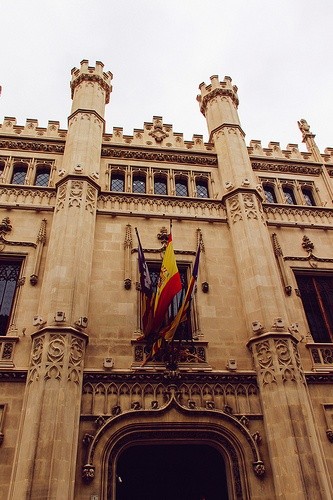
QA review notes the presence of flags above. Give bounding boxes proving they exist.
[139,239,203,372]
[143,235,185,338]
[133,231,154,310]
